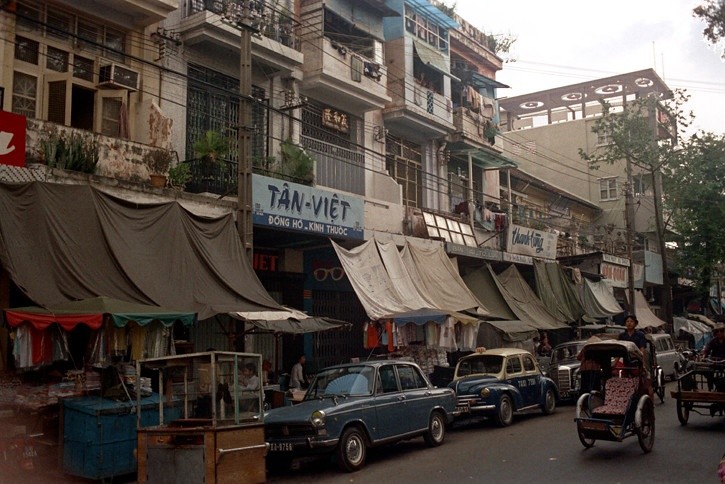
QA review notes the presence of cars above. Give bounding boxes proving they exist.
[446,347,561,427]
[648,333,683,382]
[264,360,457,473]
[542,339,587,403]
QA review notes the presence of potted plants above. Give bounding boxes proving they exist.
[143,128,239,196]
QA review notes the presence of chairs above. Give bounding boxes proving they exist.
[591,377,640,422]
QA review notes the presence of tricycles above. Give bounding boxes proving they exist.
[670,358,724,426]
[572,335,665,454]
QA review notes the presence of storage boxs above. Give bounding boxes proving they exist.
[60,391,182,479]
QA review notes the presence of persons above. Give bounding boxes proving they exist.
[243,363,260,390]
[289,353,306,389]
[533,332,553,357]
[262,360,273,385]
[703,323,725,390]
[610,314,647,368]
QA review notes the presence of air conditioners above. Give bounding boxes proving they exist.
[95,62,141,92]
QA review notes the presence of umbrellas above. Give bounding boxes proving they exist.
[2,295,194,362]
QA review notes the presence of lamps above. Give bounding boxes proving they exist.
[374,125,388,143]
[437,145,450,166]
